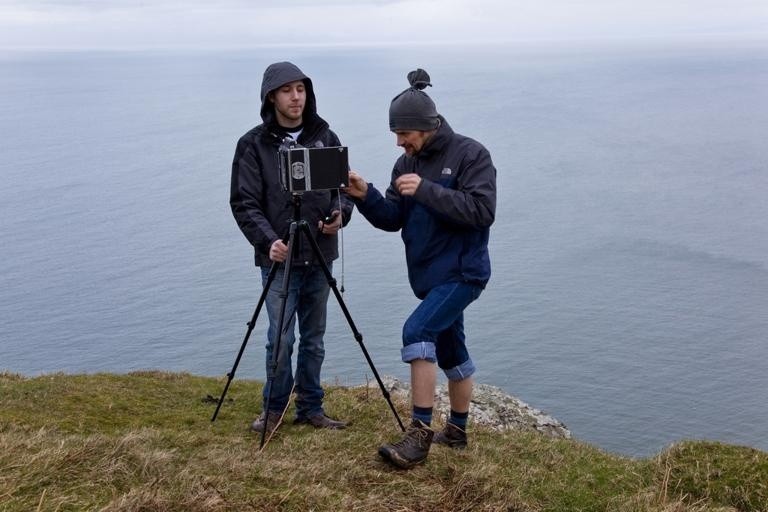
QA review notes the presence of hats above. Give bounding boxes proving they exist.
[388,68,439,131]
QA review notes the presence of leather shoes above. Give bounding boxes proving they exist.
[307,414,347,428]
[251,410,282,435]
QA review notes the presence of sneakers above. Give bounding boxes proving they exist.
[378,419,435,469]
[432,423,467,449]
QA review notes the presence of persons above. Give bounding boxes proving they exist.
[343,70,497,468]
[230,62,353,429]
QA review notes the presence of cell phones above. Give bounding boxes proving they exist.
[322,213,335,225]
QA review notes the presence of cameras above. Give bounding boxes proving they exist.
[276,136,350,193]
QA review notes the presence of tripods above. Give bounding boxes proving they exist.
[211,196,406,452]
